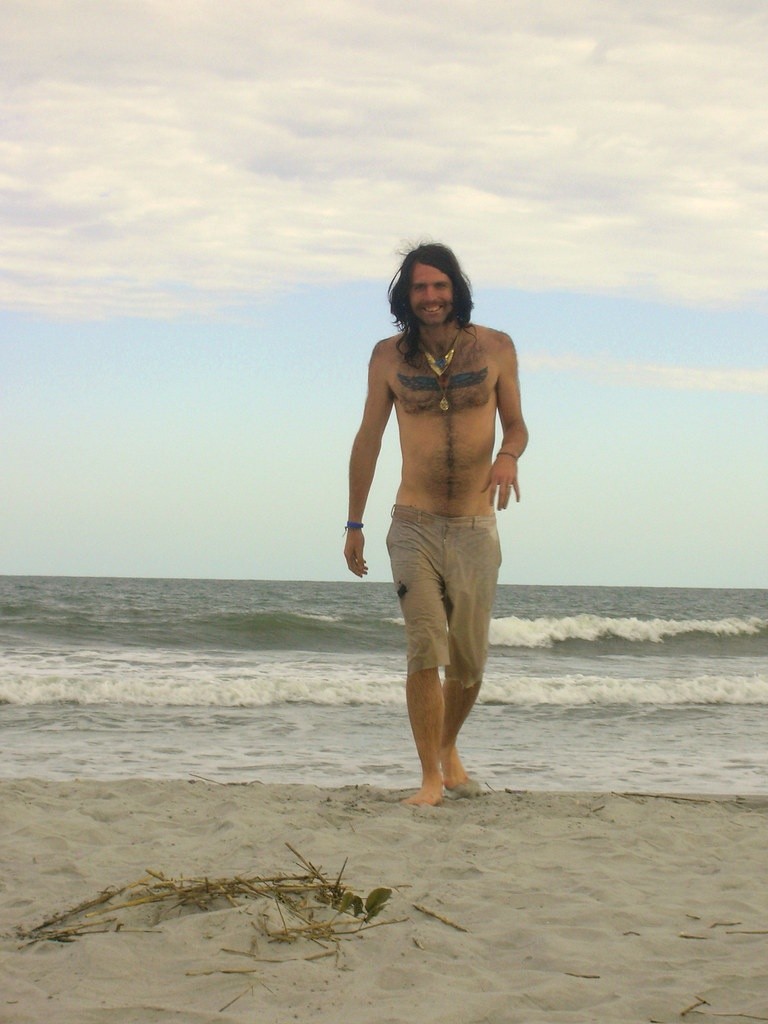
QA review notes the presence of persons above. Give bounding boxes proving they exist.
[343,247,529,806]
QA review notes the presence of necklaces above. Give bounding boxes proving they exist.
[417,327,464,411]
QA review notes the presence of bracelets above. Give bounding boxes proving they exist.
[347,521,363,529]
[497,452,517,458]
